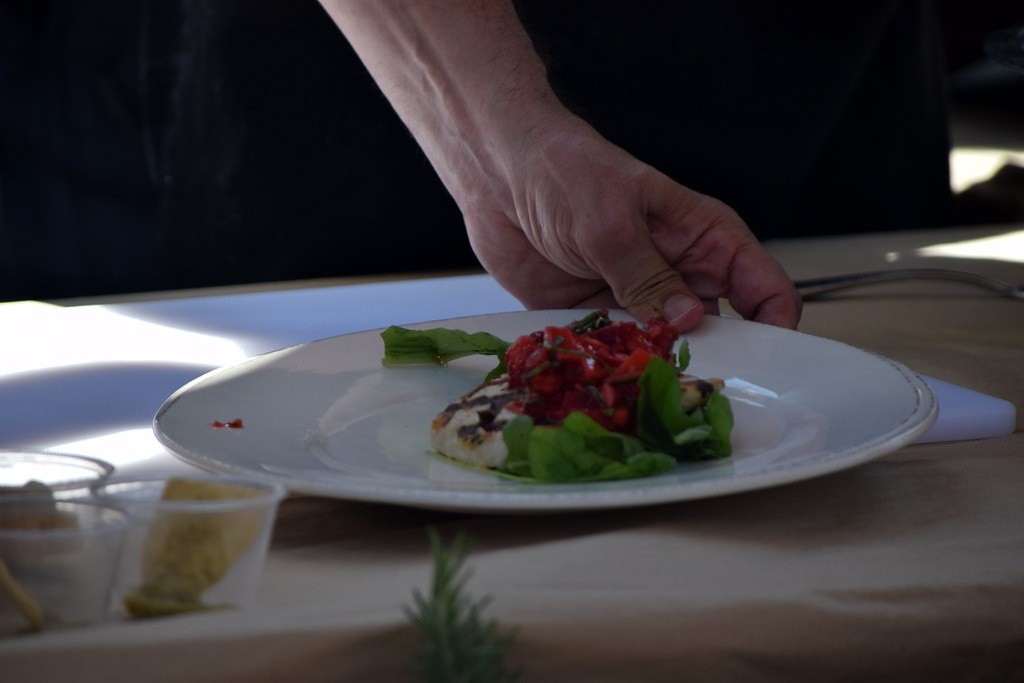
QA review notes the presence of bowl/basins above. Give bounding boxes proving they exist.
[0,447,115,510]
[0,497,130,637]
[87,478,287,617]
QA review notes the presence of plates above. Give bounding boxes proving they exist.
[152,309,940,509]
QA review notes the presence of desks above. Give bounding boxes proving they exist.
[0,224,1024,683]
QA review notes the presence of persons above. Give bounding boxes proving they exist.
[319,0,802,333]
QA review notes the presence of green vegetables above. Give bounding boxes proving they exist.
[381,325,733,482]
